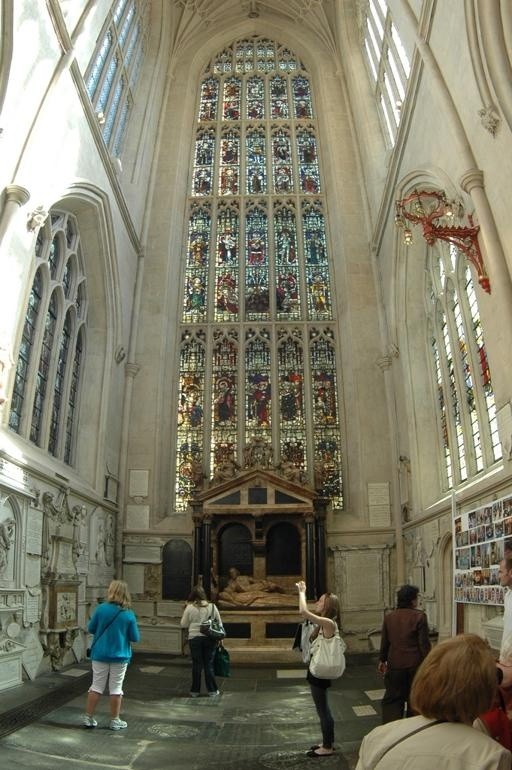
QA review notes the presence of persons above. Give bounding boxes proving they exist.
[377,583,432,724]
[293,578,344,758]
[450,496,511,607]
[354,631,511,769]
[179,585,227,699]
[494,556,511,688]
[79,580,141,731]
[221,566,300,603]
[471,684,511,753]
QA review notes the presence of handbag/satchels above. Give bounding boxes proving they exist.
[309,620,348,679]
[87,648,92,657]
[199,603,226,641]
[213,644,231,678]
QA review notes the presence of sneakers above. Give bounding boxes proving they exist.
[81,716,97,727]
[189,689,220,698]
[109,720,127,731]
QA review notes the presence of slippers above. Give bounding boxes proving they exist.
[310,743,336,751]
[307,749,333,758]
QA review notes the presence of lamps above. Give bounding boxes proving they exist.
[392,188,492,295]
